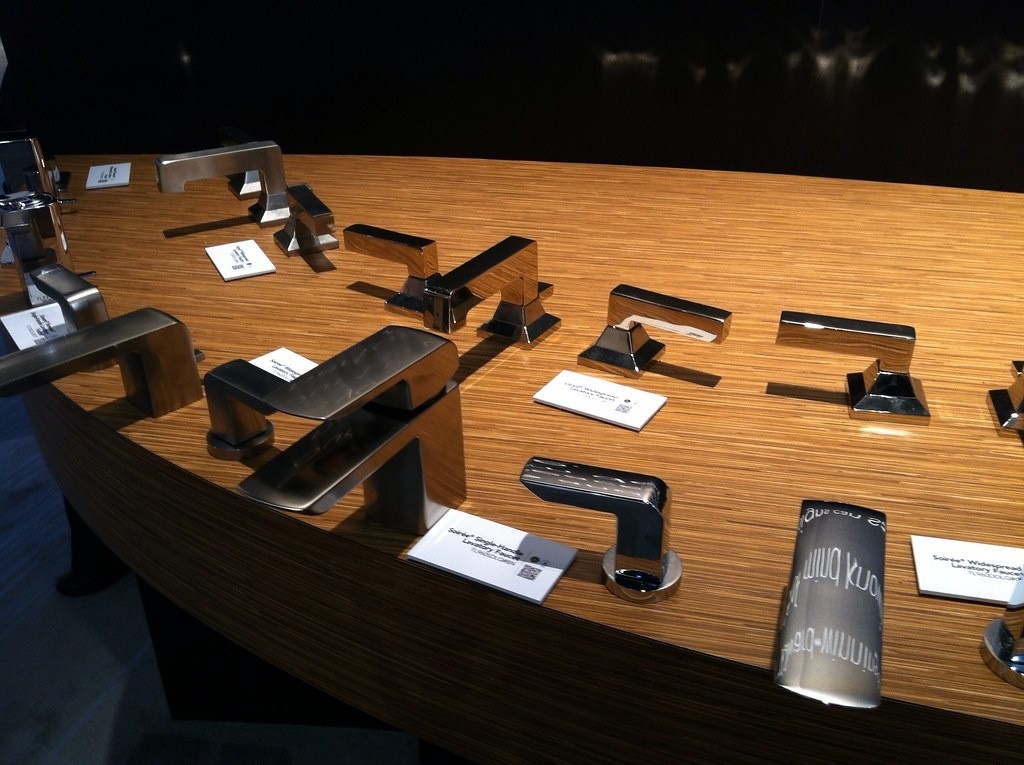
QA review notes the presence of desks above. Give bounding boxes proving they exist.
[0,148,1024,765]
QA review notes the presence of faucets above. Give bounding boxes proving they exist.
[423,233,561,347]
[578,281,735,380]
[24,266,112,332]
[0,306,204,420]
[772,312,935,424]
[0,190,76,307]
[343,223,444,321]
[770,497,886,710]
[0,129,68,264]
[231,322,468,536]
[520,454,686,606]
[153,139,290,229]
[274,184,342,258]
